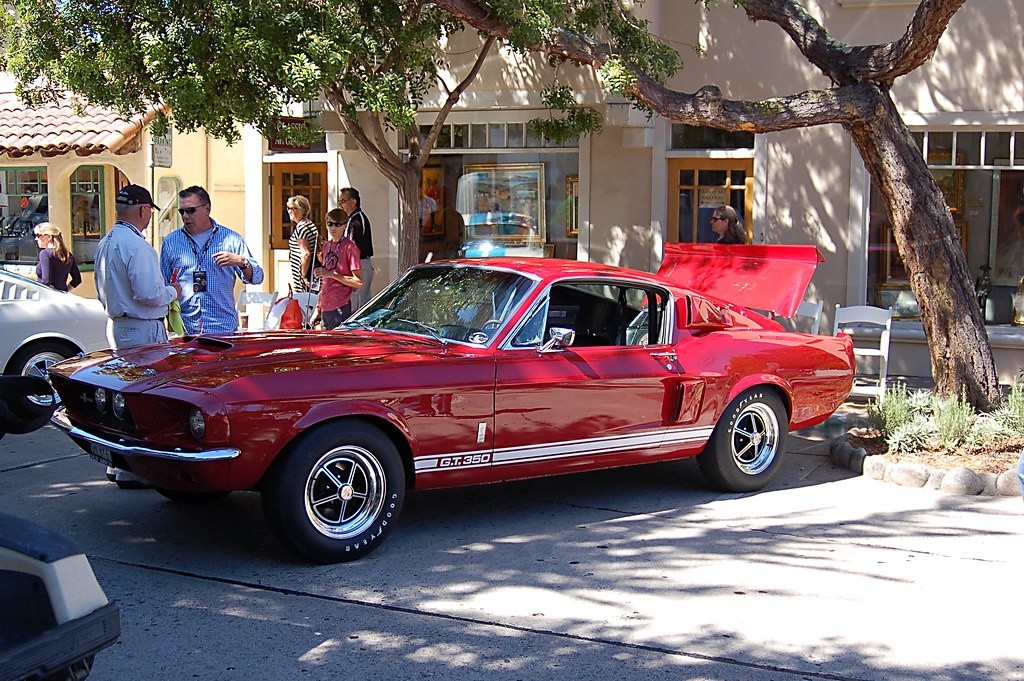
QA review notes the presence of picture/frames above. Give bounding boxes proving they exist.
[566,174,578,238]
[875,286,920,321]
[880,221,968,286]
[927,152,965,213]
[463,163,546,243]
[420,163,447,240]
[989,159,1024,286]
[462,244,554,258]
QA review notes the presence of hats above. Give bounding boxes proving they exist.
[115,184,161,211]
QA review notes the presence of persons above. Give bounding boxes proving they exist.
[161,185,264,333]
[338,186,375,310]
[310,209,364,329]
[34,222,82,292]
[94,184,182,490]
[287,196,318,317]
[711,205,747,244]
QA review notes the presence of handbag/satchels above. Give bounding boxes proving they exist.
[263,283,319,329]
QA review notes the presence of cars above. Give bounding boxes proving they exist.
[0,267,119,436]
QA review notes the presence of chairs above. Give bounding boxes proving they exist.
[235,289,320,331]
[833,303,892,408]
[769,300,823,334]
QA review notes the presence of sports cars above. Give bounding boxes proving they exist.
[45,239,858,569]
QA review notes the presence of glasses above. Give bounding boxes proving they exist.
[178,204,204,215]
[327,221,347,227]
[143,206,155,213]
[38,233,43,237]
[713,216,722,223]
[338,199,350,205]
[287,207,299,212]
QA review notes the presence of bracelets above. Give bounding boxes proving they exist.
[238,260,249,270]
